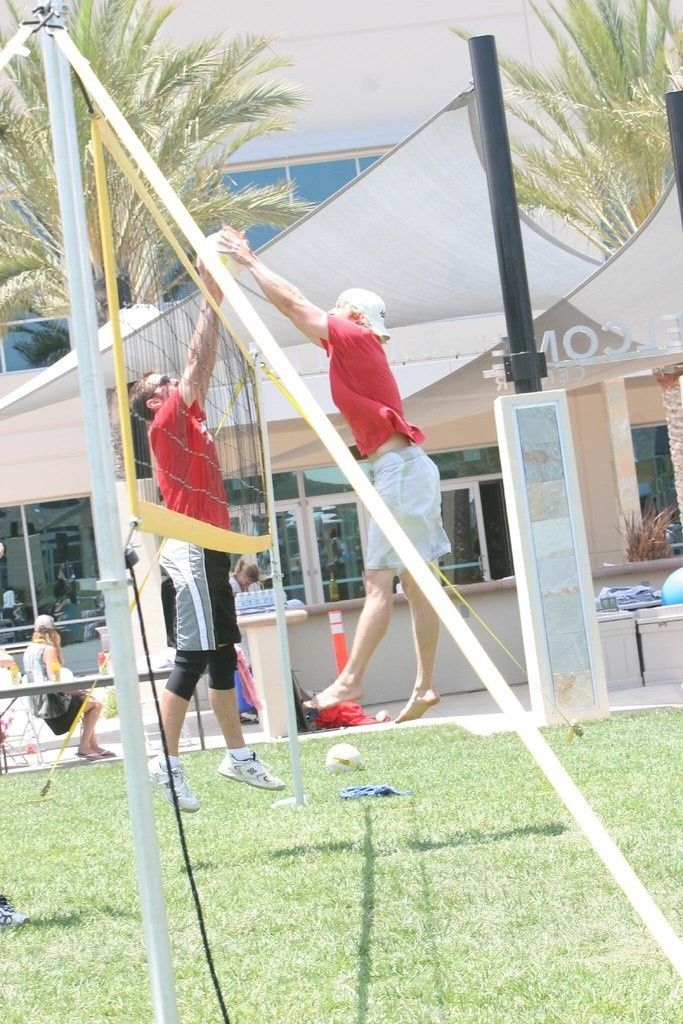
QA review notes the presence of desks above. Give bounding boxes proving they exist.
[0,667,204,774]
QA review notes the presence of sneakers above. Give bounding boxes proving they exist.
[149,755,201,813]
[217,752,286,791]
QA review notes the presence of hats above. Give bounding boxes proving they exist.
[340,287,391,341]
[34,614,55,633]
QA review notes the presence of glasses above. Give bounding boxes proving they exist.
[150,376,170,399]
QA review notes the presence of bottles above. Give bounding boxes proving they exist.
[51,659,60,682]
[98,652,112,675]
[234,589,274,606]
[11,663,18,685]
[329,569,339,601]
[33,661,43,684]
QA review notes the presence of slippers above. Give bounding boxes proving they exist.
[75,752,103,761]
[96,750,116,758]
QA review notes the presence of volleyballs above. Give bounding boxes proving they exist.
[326,742,361,776]
[201,231,248,276]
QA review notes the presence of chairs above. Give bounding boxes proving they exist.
[0,672,86,769]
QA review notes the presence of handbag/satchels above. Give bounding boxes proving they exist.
[29,646,72,720]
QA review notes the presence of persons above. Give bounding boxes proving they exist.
[3,561,81,639]
[24,614,118,760]
[229,558,260,723]
[127,255,288,813]
[326,528,350,599]
[216,224,451,724]
